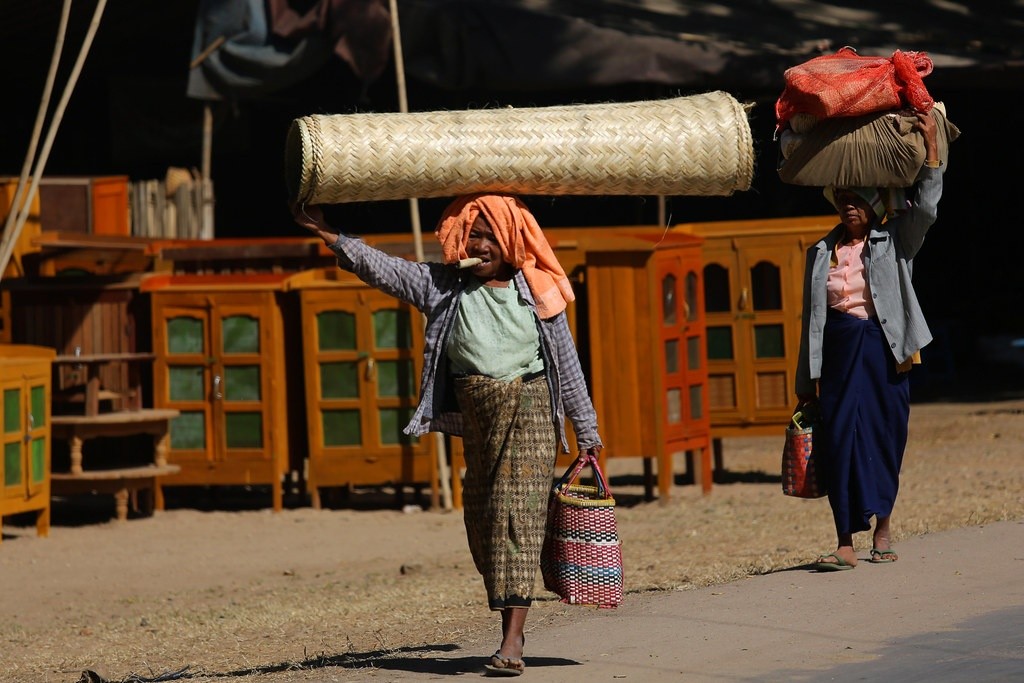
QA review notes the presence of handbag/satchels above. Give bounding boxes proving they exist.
[541,456,622,606]
[782,400,829,498]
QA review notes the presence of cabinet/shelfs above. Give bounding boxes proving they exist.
[140,214,843,516]
[0,344,55,546]
[47,352,181,527]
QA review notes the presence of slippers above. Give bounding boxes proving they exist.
[815,553,855,570]
[489,649,525,675]
[869,549,896,562]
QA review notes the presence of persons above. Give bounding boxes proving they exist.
[297,193,605,676]
[795,108,942,570]
